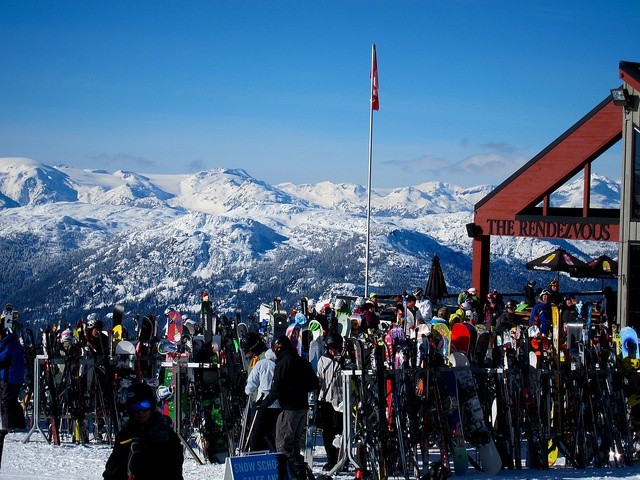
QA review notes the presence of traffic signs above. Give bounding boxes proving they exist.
[224,450,291,480]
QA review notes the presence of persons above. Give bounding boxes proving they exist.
[309,282,518,340]
[315,333,350,472]
[600,286,618,331]
[102,383,184,479]
[41,279,103,448]
[240,331,268,452]
[527,289,550,336]
[1,303,26,467]
[258,335,318,480]
[548,281,560,309]
[559,297,578,348]
[409,318,428,446]
[245,349,280,452]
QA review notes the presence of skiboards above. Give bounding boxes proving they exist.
[348,294,638,479]
[36,303,132,448]
[30,289,348,446]
[347,295,454,479]
[198,291,349,474]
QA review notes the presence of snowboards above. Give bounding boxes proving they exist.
[431,301,638,478]
[135,307,200,438]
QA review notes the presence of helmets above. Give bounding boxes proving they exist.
[505,301,516,308]
[538,288,551,298]
[126,383,153,412]
[413,287,423,296]
[181,314,189,321]
[335,299,345,312]
[438,306,448,318]
[6,303,14,310]
[164,308,174,316]
[564,294,575,300]
[86,318,103,329]
[354,297,366,308]
[272,336,291,357]
[324,333,343,348]
[240,332,262,353]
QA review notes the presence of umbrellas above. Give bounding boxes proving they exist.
[526,247,587,298]
[425,252,448,303]
[570,255,618,301]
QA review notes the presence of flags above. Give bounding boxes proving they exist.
[369,43,379,111]
